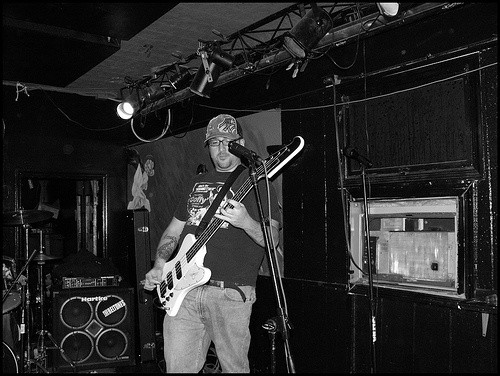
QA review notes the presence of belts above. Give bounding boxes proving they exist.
[207,279,250,302]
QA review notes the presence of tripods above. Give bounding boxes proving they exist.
[29,229,79,376]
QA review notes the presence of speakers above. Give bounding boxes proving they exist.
[46,286,138,371]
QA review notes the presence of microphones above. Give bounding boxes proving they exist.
[228,142,264,163]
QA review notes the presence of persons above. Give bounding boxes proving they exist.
[143,113,283,374]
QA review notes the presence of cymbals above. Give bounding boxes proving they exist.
[0,211,54,225]
[16,254,63,261]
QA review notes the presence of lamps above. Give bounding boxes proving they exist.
[115,93,143,120]
[283,5,335,61]
[194,47,235,99]
[378,3,402,17]
[169,68,193,91]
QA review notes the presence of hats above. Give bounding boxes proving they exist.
[203,114,243,143]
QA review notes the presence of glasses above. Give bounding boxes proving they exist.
[208,136,244,146]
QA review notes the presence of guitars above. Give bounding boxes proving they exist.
[156,136,305,317]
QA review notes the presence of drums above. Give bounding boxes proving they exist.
[2,256,22,314]
[2,341,19,374]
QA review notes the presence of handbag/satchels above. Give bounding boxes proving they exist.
[55,248,119,278]
[31,264,65,298]
